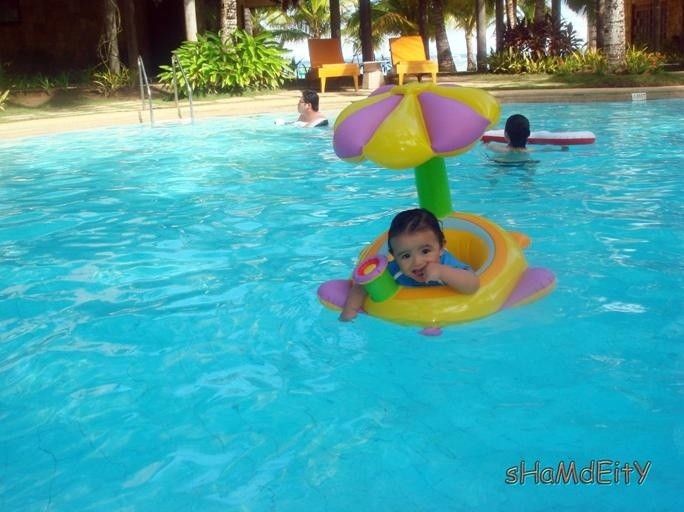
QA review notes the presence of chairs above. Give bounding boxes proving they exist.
[306,36,360,94]
[388,35,439,85]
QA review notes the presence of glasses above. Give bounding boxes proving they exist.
[299,100,310,103]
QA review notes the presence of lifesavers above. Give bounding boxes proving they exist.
[318,82,554,336]
[482,129,595,145]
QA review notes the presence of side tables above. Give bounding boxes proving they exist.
[361,59,389,91]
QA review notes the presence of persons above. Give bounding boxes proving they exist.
[488,114,530,152]
[338,208,479,322]
[297,90,327,127]
